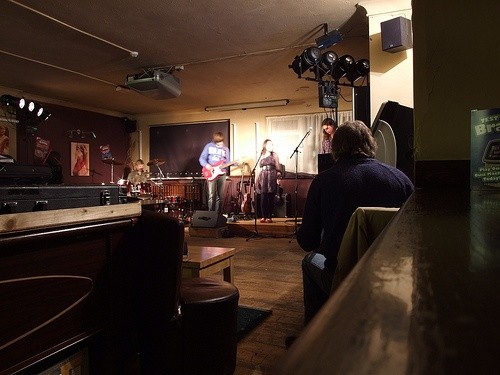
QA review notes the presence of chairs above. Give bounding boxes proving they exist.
[332,206,399,289]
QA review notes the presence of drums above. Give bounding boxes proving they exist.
[166,195,180,204]
[117,179,132,197]
[138,182,152,199]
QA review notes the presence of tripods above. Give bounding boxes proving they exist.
[245,148,276,242]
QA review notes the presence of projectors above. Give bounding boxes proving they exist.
[124,67,182,100]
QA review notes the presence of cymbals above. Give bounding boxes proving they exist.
[101,158,123,165]
[146,158,167,167]
[129,171,152,175]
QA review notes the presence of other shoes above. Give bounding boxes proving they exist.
[285,337,296,348]
[260,219,266,223]
[267,220,272,223]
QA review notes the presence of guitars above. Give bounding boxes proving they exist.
[241,170,252,215]
[202,157,243,180]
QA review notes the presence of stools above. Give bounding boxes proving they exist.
[177,276,239,375]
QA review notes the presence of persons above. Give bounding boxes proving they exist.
[73,143,88,175]
[0,126,9,157]
[254,139,283,223]
[127,158,148,184]
[284,120,415,348]
[320,117,339,155]
[199,131,240,212]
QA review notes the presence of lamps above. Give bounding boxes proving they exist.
[0,94,51,120]
[291,46,321,73]
[331,55,355,81]
[346,58,370,83]
[205,99,289,111]
[310,51,338,79]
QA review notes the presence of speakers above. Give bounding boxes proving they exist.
[381,16,412,53]
[274,193,291,218]
[190,210,227,228]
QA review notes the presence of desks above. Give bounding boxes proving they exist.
[182,245,236,287]
